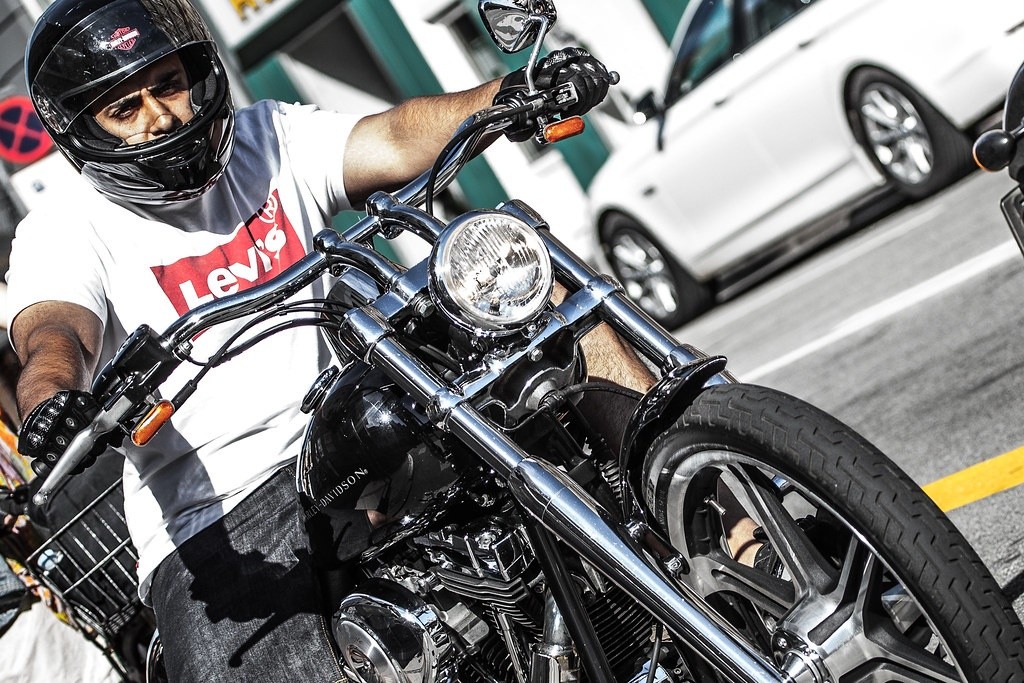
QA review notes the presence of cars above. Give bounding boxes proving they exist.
[583,0,1024,333]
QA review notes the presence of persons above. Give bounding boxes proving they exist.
[0,556,126,682]
[4,0,806,683]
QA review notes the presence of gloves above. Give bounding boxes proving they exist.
[18,388,95,464]
[498,47,608,135]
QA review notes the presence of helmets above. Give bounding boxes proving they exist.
[25,5,235,214]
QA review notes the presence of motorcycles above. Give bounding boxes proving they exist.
[0,0,1024,683]
